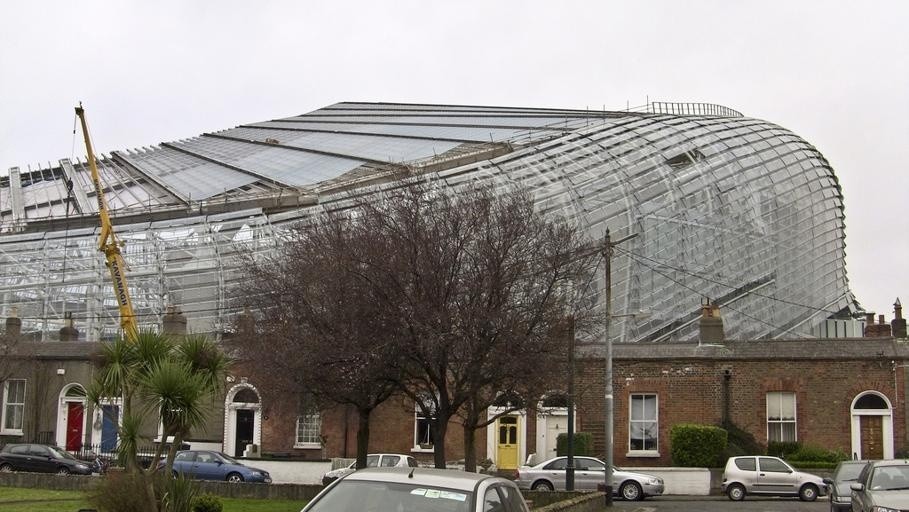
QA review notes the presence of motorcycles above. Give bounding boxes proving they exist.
[89,450,106,476]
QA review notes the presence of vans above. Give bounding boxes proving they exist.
[0,442,91,477]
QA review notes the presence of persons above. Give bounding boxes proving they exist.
[91,455,103,471]
[87,446,97,462]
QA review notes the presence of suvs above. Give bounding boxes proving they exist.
[322,451,417,487]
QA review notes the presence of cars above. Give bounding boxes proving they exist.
[516,453,664,501]
[852,457,909,511]
[300,465,531,511]
[157,449,273,483]
[824,459,876,510]
[721,456,828,500]
